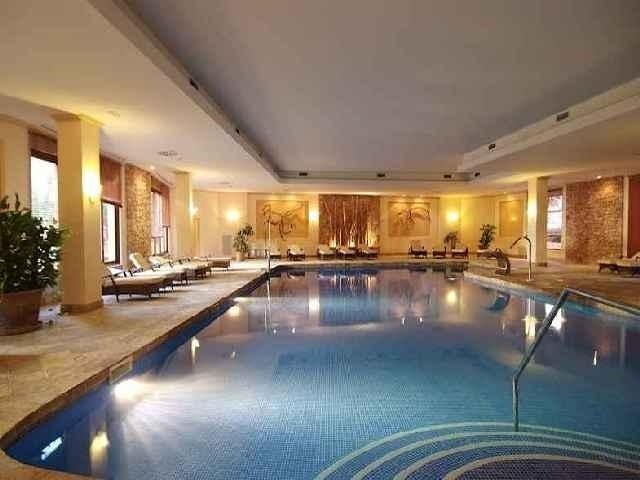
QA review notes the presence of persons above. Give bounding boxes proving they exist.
[490,248,510,274]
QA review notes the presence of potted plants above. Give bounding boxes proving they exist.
[477,223,497,250]
[233,224,255,263]
[0,190,73,336]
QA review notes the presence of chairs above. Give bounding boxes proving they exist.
[263,238,503,259]
[616,250,639,274]
[593,249,621,271]
[100,248,233,301]
